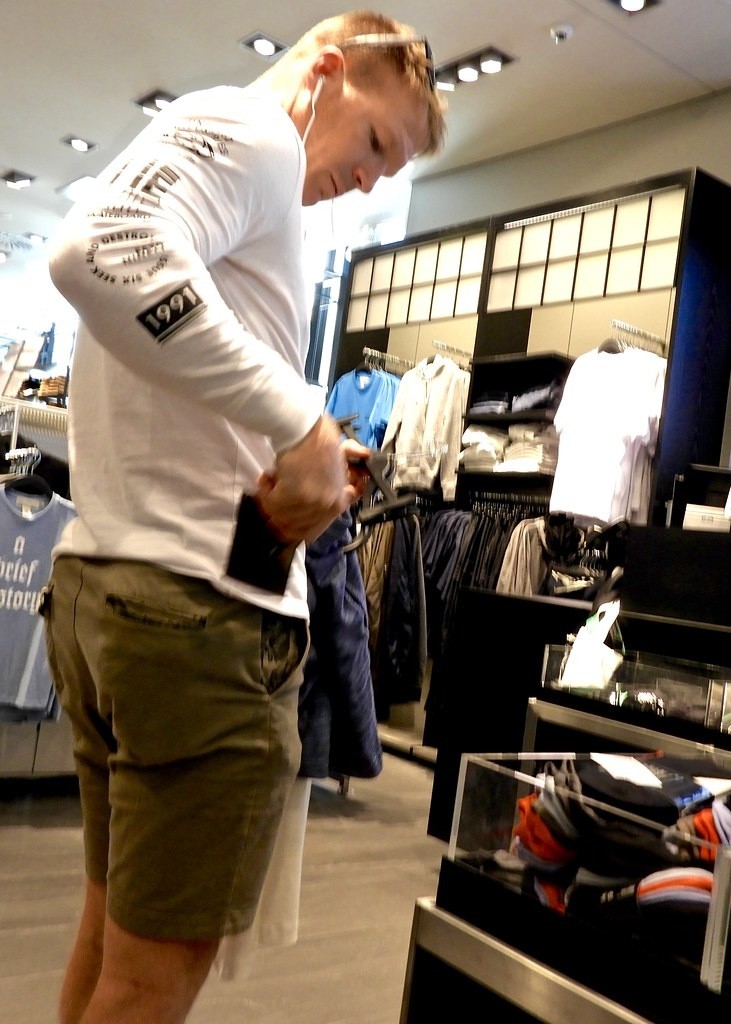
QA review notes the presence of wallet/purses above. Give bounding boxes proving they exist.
[226,492,298,593]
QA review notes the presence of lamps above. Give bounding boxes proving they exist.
[436,49,502,92]
[253,34,277,58]
[142,93,174,118]
[6,172,33,191]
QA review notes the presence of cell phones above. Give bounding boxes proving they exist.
[224,490,297,596]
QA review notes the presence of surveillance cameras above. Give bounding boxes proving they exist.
[551,23,574,43]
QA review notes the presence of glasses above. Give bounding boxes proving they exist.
[336,32,437,92]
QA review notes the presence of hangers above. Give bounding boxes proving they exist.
[0,447,85,526]
[329,319,670,540]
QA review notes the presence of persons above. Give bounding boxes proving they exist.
[40,10,446,1024]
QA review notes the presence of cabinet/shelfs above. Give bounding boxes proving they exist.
[327,167,731,504]
